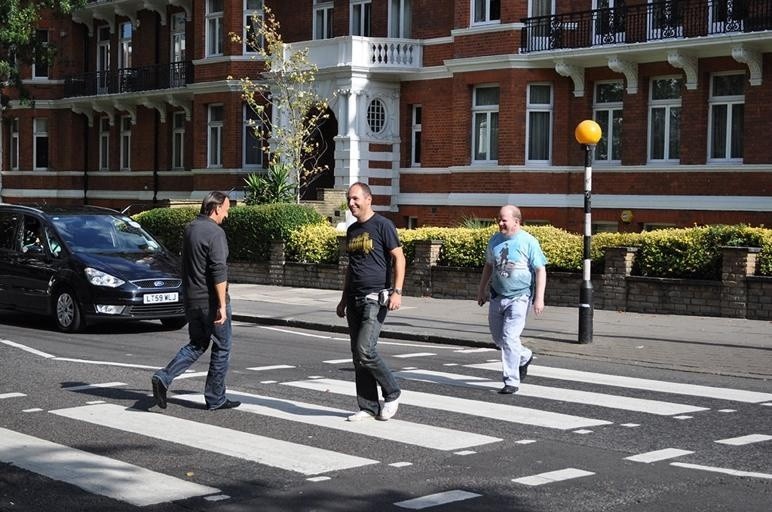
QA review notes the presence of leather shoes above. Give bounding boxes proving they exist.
[215,399,241,409]
[152,376,167,409]
[519,354,533,380]
[500,384,519,394]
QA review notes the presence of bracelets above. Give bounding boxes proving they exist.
[394,288,402,295]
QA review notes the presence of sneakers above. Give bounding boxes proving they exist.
[348,410,380,421]
[380,393,401,420]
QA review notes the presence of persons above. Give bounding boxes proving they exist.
[478,205,547,393]
[336,182,406,420]
[152,192,250,410]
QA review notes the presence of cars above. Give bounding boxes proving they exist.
[0,202,190,333]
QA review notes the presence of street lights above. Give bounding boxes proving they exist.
[575,119,602,344]
